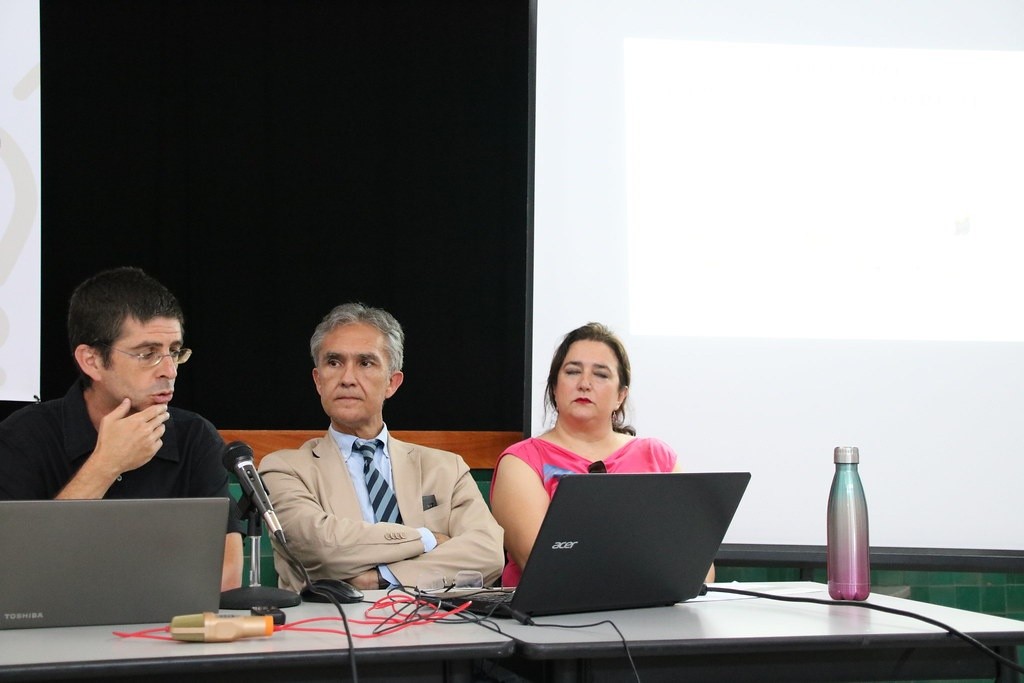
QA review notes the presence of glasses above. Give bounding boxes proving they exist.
[588,460,607,473]
[89,341,192,367]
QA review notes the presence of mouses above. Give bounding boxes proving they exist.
[300,578,364,604]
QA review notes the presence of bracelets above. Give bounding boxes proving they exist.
[376,567,390,590]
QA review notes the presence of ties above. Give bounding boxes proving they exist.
[351,439,403,525]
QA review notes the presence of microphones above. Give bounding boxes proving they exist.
[222,441,285,545]
[169,613,274,644]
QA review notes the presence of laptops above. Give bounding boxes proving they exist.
[414,472,753,615]
[0,497,231,631]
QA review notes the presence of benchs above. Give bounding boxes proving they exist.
[218,430,524,586]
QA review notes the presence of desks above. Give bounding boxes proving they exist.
[0,580,1024,683]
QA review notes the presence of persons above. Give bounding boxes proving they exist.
[257,301,504,590]
[0,266,249,592]
[491,322,716,585]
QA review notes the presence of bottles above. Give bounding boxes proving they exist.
[827,447,871,602]
